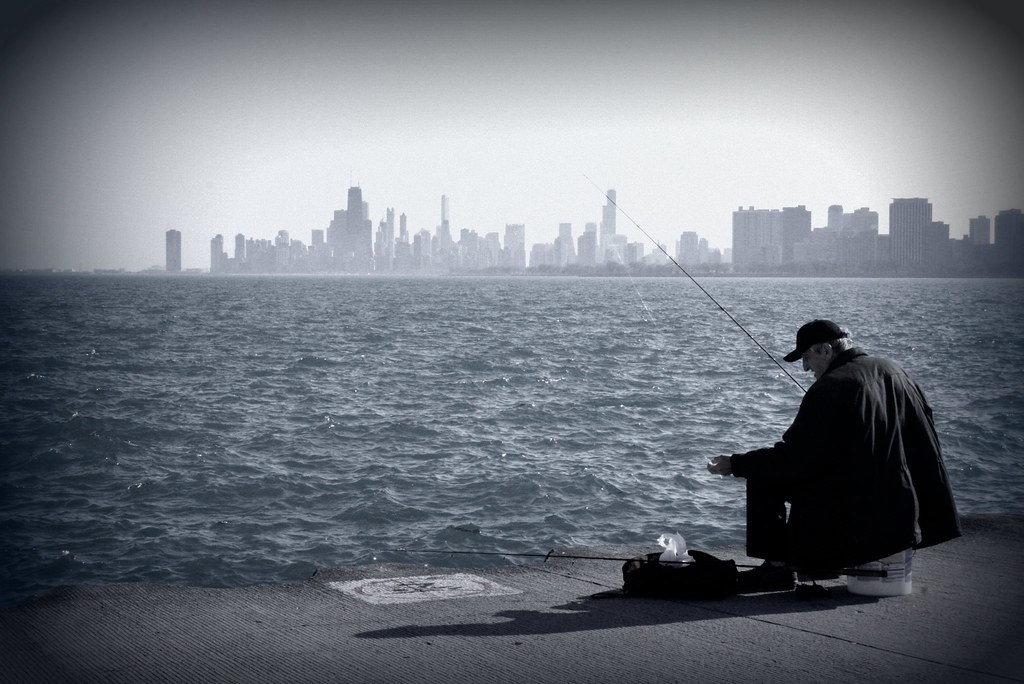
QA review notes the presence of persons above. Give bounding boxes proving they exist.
[707,319,962,594]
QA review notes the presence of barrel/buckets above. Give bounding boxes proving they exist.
[848,547,917,596]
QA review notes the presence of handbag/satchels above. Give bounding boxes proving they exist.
[621,550,740,600]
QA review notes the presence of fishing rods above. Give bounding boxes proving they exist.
[395,546,889,578]
[597,186,808,393]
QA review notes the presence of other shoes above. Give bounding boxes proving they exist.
[733,557,795,593]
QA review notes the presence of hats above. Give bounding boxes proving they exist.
[783,319,847,362]
